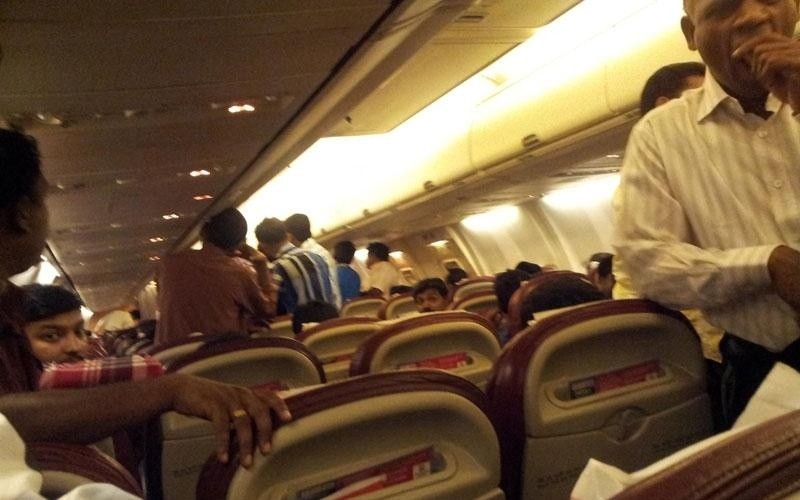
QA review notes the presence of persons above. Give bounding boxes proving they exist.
[1,123,411,500]
[493,269,534,350]
[595,256,616,297]
[611,60,725,429]
[518,261,542,275]
[447,267,469,302]
[413,277,451,314]
[612,0,800,434]
